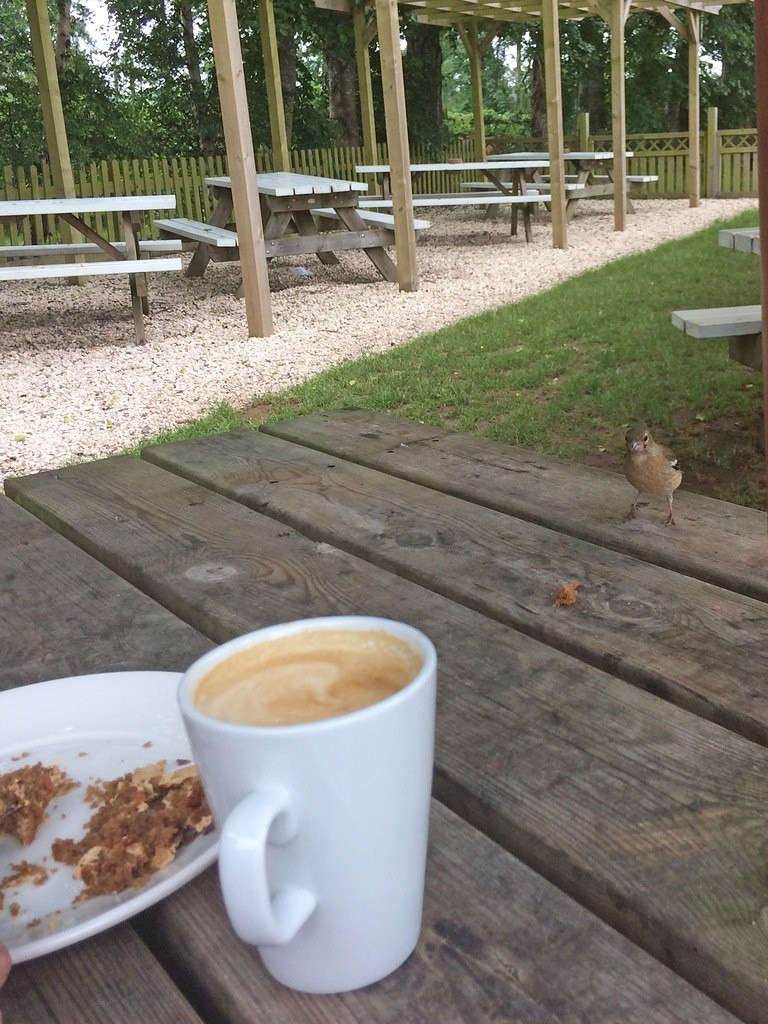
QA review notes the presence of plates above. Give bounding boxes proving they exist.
[0,668,220,966]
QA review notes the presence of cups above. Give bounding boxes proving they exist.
[176,614,438,996]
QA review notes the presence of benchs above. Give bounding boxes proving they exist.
[358,189,539,235]
[152,218,245,298]
[460,182,585,224]
[0,240,182,314]
[357,193,551,242]
[540,175,659,214]
[310,208,430,282]
[0,257,182,347]
[671,305,763,375]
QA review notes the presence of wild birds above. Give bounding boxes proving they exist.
[623,427,683,527]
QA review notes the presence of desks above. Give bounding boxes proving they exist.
[0,194,177,346]
[0,415,768,1024]
[482,152,637,223]
[354,160,550,243]
[717,228,762,254]
[185,172,403,297]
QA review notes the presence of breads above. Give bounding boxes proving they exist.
[0,763,210,894]
